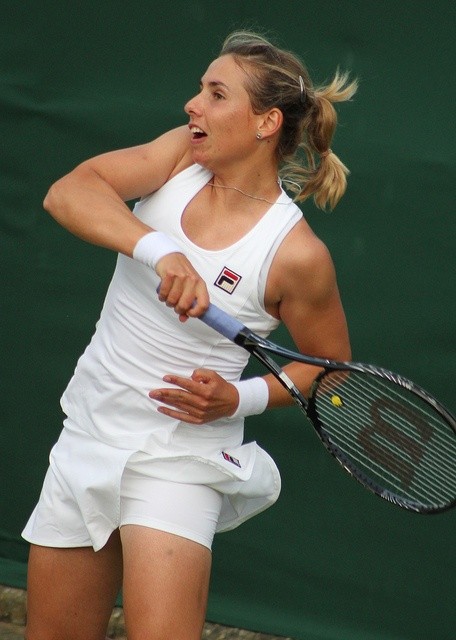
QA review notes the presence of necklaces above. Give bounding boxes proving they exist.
[207,179,302,206]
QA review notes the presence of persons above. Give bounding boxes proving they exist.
[21,30,360,639]
